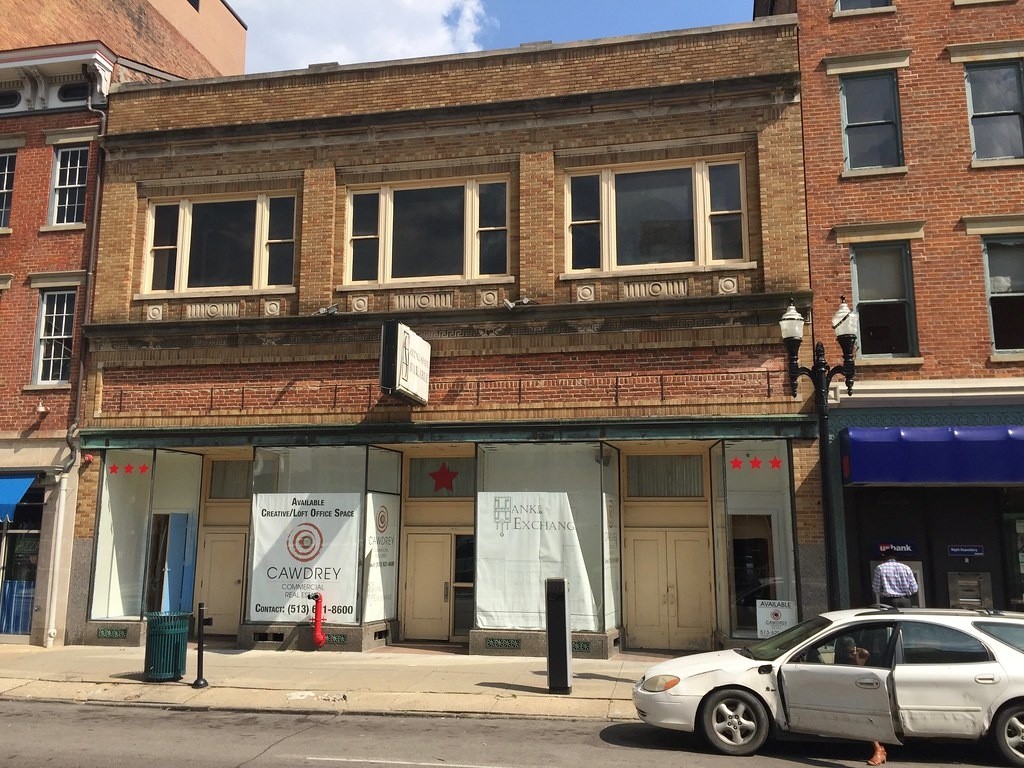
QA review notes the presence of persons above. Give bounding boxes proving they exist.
[873,547,918,609]
[832,635,889,766]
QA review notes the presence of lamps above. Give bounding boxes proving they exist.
[34,402,51,414]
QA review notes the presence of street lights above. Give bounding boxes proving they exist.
[779,293,860,612]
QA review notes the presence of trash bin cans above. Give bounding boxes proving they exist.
[144,611,194,681]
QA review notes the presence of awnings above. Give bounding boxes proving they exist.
[840,422,1024,488]
[0,470,48,523]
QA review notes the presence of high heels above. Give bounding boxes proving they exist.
[867,747,886,766]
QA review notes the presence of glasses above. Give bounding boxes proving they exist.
[845,646,856,657]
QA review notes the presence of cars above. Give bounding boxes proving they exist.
[631,601,1024,768]
